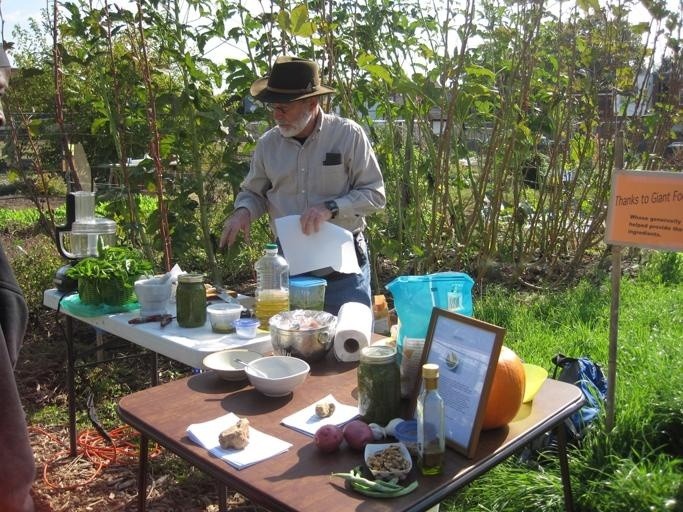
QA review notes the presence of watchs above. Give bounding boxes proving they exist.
[325,199,338,220]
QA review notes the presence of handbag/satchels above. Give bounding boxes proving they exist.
[518,354,609,465]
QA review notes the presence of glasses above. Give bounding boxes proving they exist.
[266,100,305,114]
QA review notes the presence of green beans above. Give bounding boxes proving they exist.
[331,465,419,498]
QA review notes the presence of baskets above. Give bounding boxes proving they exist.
[77,277,139,306]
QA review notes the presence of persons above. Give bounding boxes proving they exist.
[218,57,386,315]
[0,43,35,512]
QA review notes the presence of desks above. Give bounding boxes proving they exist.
[39,271,331,459]
[112,324,586,512]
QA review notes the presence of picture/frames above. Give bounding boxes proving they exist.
[402,305,505,460]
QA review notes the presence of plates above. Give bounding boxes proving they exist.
[363,442,413,482]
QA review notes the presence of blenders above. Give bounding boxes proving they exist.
[54,190,118,295]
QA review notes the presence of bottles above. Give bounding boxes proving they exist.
[415,362,446,477]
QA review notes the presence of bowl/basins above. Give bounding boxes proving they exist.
[205,303,246,332]
[232,317,260,341]
[267,310,336,365]
[133,279,172,302]
[393,419,437,457]
[244,356,311,399]
[202,350,261,381]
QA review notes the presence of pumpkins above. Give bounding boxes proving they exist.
[481,345,526,431]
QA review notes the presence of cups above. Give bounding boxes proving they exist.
[137,295,168,322]
[521,363,548,404]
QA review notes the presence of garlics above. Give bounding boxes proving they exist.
[385,418,405,437]
[368,422,388,441]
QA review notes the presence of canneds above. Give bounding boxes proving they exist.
[356,345,402,426]
[177,274,207,327]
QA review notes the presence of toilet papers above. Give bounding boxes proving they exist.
[331,302,375,365]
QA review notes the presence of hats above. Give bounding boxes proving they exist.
[250,56,337,104]
